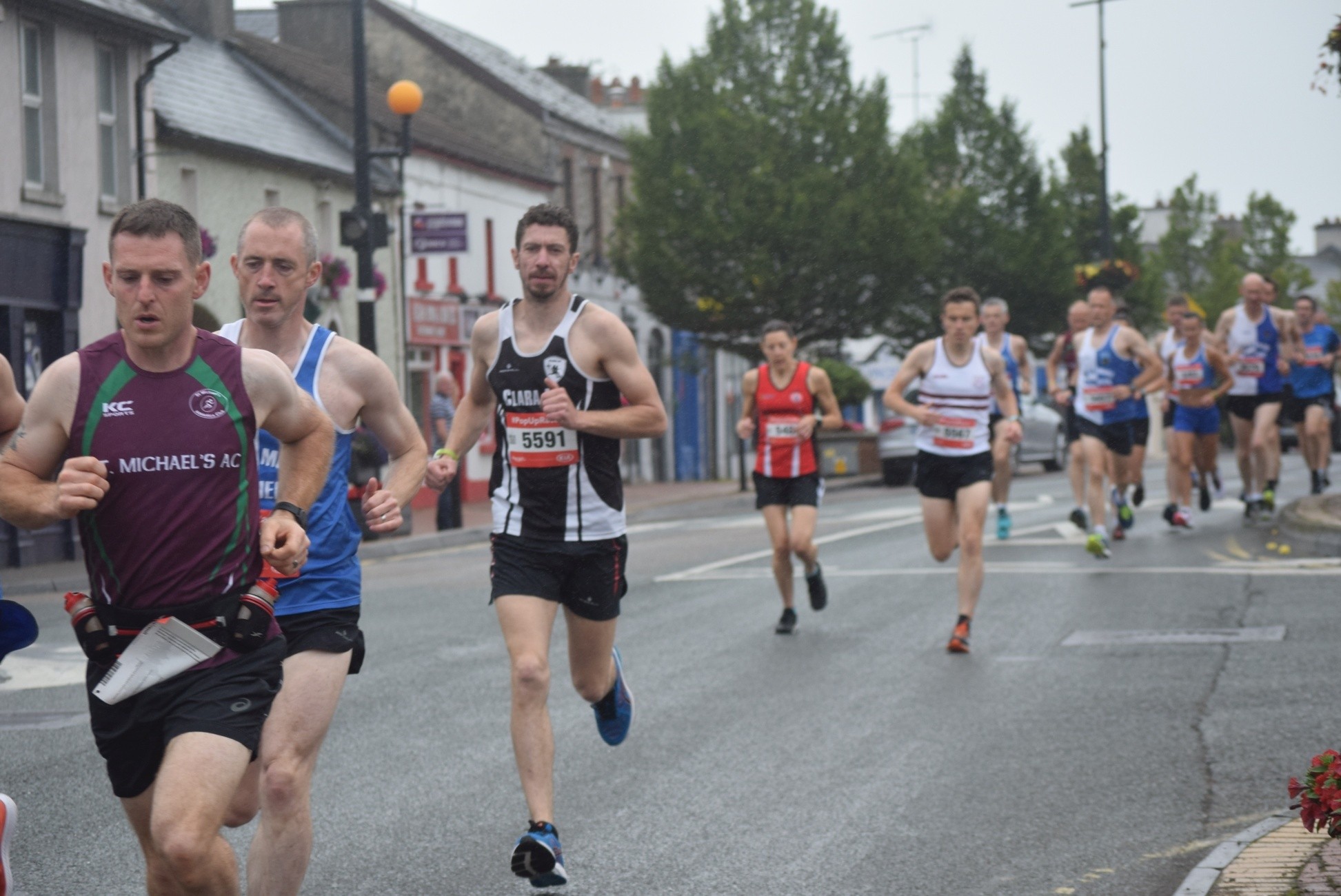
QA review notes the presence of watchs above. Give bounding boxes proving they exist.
[272,500,309,530]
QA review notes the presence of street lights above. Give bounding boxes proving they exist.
[350,78,425,356]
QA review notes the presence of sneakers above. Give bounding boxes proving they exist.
[590,647,634,746]
[0,793,17,896]
[510,820,568,887]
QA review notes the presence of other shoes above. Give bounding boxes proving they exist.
[948,621,970,652]
[1239,469,1331,522]
[1113,528,1123,538]
[1173,506,1194,528]
[1087,534,1111,558]
[1131,489,1144,505]
[1112,487,1132,528]
[806,561,827,609]
[775,609,797,634]
[1200,489,1210,510]
[1191,472,1200,486]
[995,510,1011,539]
[1213,473,1221,490]
[1070,509,1087,530]
[1163,504,1178,524]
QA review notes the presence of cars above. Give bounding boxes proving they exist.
[874,386,1070,488]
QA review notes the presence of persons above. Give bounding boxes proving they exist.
[1070,288,1166,559]
[974,297,1034,543]
[1045,271,1341,540]
[0,198,339,895]
[211,207,430,896]
[734,319,846,636]
[424,201,671,890]
[883,287,1025,655]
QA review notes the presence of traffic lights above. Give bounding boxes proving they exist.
[337,209,390,250]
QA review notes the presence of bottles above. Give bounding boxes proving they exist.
[232,577,279,650]
[63,591,112,661]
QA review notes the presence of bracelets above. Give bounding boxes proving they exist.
[1007,416,1024,424]
[1125,381,1137,394]
[432,447,459,460]
[814,416,823,430]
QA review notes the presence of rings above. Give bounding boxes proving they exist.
[292,559,299,568]
[381,514,387,523]
